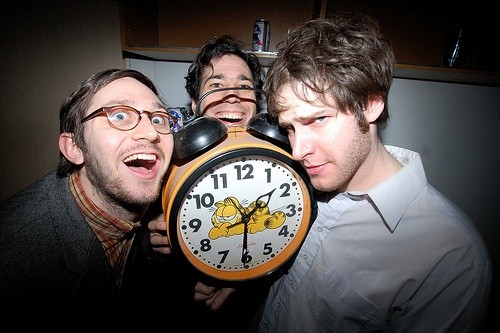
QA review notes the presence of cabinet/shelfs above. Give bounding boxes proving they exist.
[119,0,500,86]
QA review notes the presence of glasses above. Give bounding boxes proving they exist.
[82,103,179,133]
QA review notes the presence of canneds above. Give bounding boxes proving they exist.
[167,106,184,134]
[252,19,270,52]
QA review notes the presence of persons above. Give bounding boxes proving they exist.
[0,67,180,333]
[131,33,285,333]
[244,13,493,333]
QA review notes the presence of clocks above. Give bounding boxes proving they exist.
[162,87,317,286]
